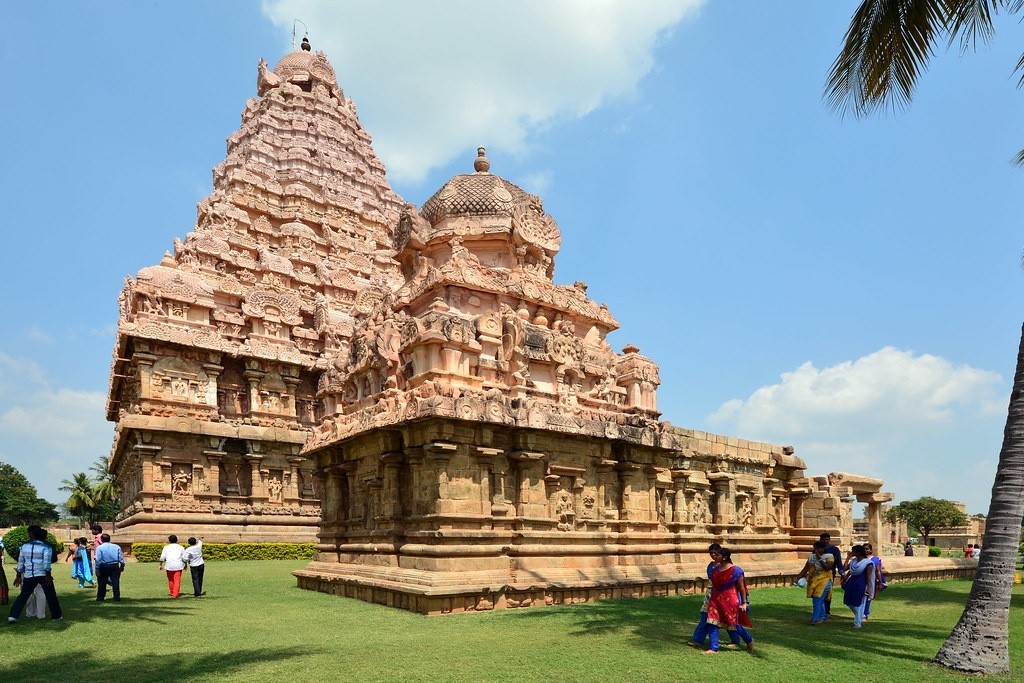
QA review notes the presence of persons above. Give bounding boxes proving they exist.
[159,534,187,598]
[840,544,875,630]
[804,541,833,626]
[182,536,205,597]
[71,537,96,589]
[904,542,913,556]
[947,548,952,555]
[8,525,63,621]
[95,534,125,602]
[688,543,722,646]
[963,544,973,558]
[65,538,79,562]
[701,548,753,654]
[841,542,888,622]
[793,533,847,617]
[91,525,112,586]
[135,287,752,524]
[970,544,981,557]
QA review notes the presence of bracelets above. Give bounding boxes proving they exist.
[842,573,844,576]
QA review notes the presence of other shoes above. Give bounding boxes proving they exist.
[8,617,17,622]
[52,616,64,621]
[853,624,860,629]
[809,620,815,625]
[817,620,825,623]
